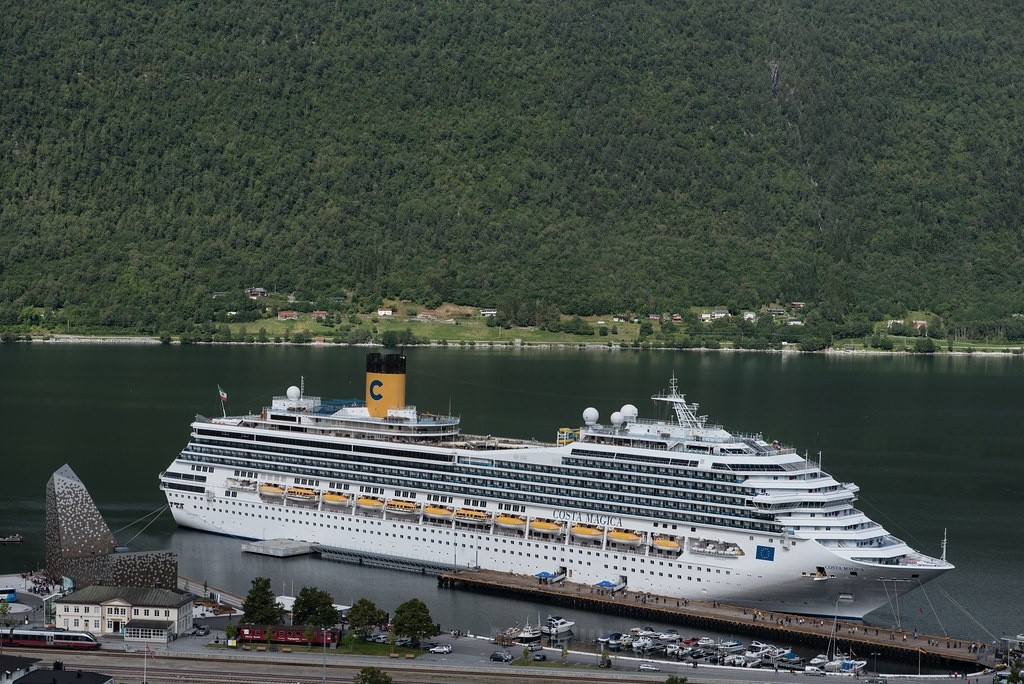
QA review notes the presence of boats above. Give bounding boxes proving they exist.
[453,508,489,526]
[385,499,417,515]
[530,519,560,535]
[540,614,575,634]
[0,534,23,543]
[606,528,641,545]
[494,515,526,531]
[516,614,542,642]
[652,535,683,552]
[286,487,318,503]
[596,596,868,674]
[322,492,350,506]
[259,485,284,498]
[570,526,605,541]
[424,504,453,520]
[356,496,382,511]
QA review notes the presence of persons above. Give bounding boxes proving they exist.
[29,578,57,595]
[947,669,981,684]
[992,672,1000,684]
[451,628,459,640]
[536,576,691,608]
[749,606,987,654]
[712,599,721,608]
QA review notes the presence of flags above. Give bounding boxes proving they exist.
[219,387,227,401]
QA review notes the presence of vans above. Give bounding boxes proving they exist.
[804,666,827,676]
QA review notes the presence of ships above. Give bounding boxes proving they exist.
[153,352,954,624]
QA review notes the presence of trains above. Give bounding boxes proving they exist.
[0,626,101,652]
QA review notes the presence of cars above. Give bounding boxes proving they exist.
[535,653,546,660]
[489,650,513,663]
[353,630,452,651]
[638,664,659,673]
[528,644,544,652]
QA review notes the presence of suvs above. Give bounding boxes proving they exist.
[196,625,210,636]
[429,645,450,655]
